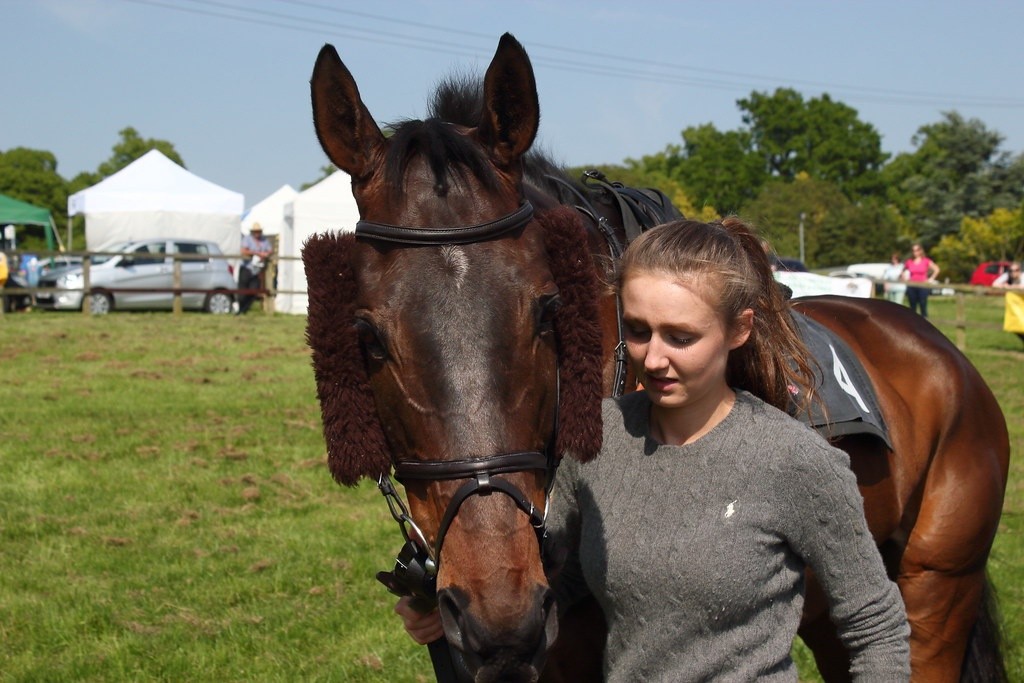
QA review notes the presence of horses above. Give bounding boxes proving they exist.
[311,31,1011,683]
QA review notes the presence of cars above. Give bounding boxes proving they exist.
[51,237,237,315]
[971,262,1013,294]
[35,255,103,279]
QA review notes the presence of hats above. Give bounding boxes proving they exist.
[251,223,265,230]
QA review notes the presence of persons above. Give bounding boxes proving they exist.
[992,261,1024,341]
[238,222,273,314]
[882,254,907,304]
[395,215,911,683]
[900,244,940,317]
